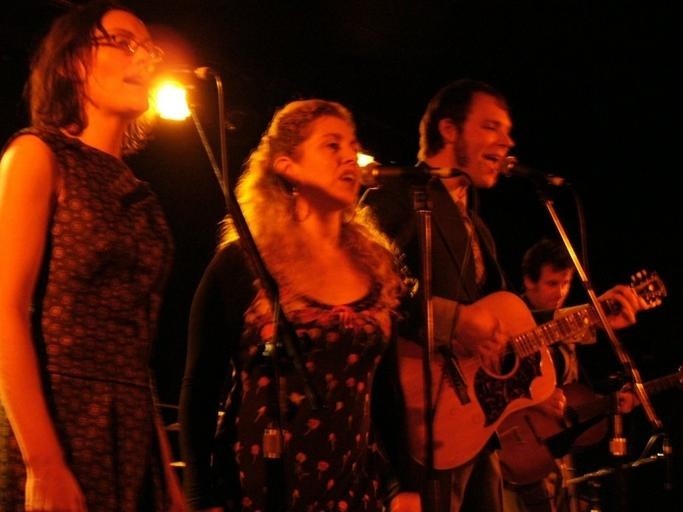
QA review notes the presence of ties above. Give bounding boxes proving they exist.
[455,198,486,289]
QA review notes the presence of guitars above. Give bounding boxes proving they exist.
[396,268,669,471]
[492,364,682,493]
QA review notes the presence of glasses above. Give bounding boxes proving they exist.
[72,33,166,65]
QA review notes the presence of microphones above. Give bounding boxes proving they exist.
[140,61,209,82]
[496,154,564,187]
[359,160,463,182]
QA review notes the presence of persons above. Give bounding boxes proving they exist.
[517,239,641,512]
[0,0,189,512]
[180,101,423,512]
[360,83,648,512]
